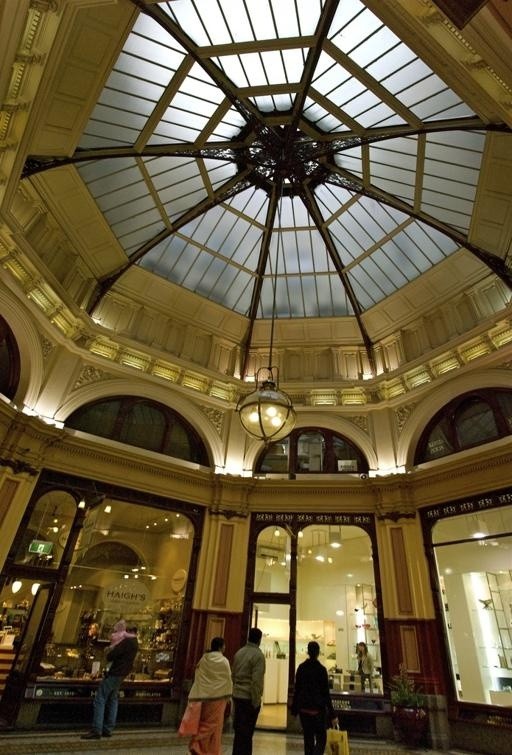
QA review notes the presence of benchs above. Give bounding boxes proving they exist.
[334,706,392,739]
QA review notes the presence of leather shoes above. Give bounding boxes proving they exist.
[81,731,111,739]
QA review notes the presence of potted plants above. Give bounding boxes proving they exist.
[384,661,429,749]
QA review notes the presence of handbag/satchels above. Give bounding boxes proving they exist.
[325,727,349,755]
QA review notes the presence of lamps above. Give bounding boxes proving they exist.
[233,174,299,447]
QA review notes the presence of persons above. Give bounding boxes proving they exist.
[230,627,267,754]
[357,643,375,693]
[291,641,337,754]
[76,621,100,677]
[80,627,137,739]
[101,619,136,672]
[184,637,234,755]
